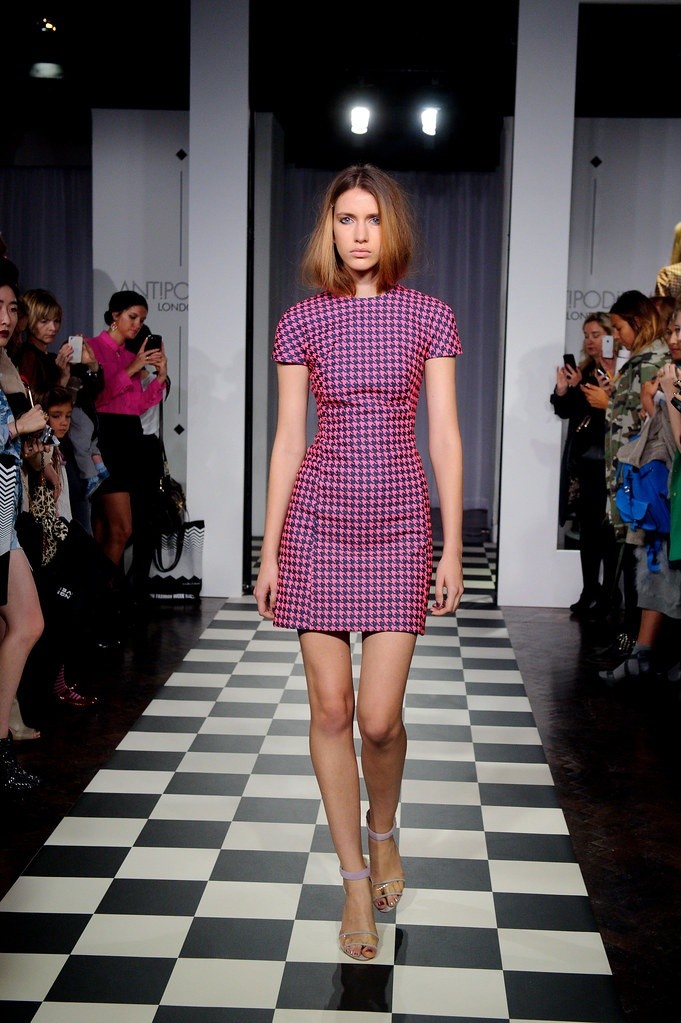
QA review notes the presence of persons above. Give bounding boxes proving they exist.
[253,166,464,962]
[0,279,167,792]
[577,290,681,687]
[549,312,610,614]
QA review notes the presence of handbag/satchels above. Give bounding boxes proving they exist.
[152,477,186,572]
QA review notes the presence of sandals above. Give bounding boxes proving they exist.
[654,662,681,686]
[598,647,651,688]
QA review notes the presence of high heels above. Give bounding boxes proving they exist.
[340,856,379,961]
[366,808,405,912]
[569,582,624,617]
[8,696,40,741]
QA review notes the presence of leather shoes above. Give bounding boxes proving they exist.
[48,686,99,710]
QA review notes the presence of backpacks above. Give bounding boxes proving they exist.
[614,433,671,572]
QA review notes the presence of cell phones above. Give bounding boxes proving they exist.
[597,369,609,380]
[601,335,614,359]
[68,335,83,365]
[563,355,577,379]
[147,334,163,351]
